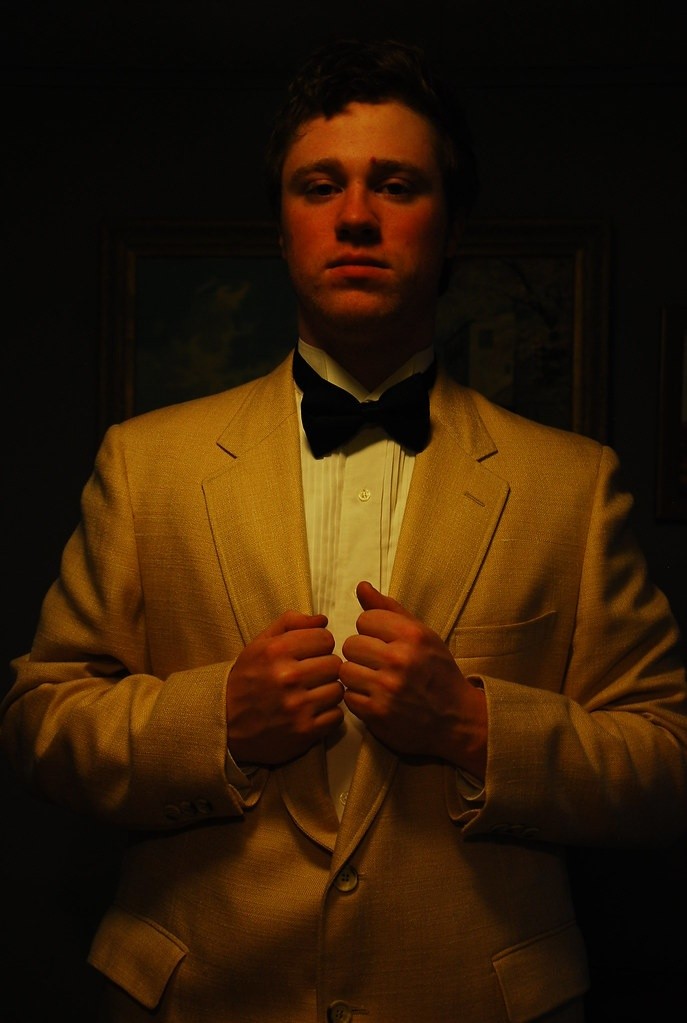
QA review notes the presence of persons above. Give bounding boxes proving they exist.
[2,31,686,1023]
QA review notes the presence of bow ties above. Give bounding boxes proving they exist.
[296,354,439,457]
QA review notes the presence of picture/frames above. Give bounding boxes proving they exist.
[649,302,686,526]
[95,205,616,463]
